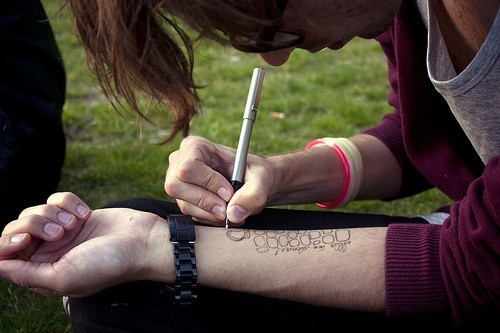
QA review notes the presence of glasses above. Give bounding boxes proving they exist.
[227,0,303,53]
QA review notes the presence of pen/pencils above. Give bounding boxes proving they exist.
[224,66,266,231]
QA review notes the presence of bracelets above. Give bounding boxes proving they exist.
[305,137,365,210]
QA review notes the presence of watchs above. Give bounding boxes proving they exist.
[155,213,201,306]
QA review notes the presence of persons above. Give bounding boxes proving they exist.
[1,0,500,333]
[1,0,67,235]
